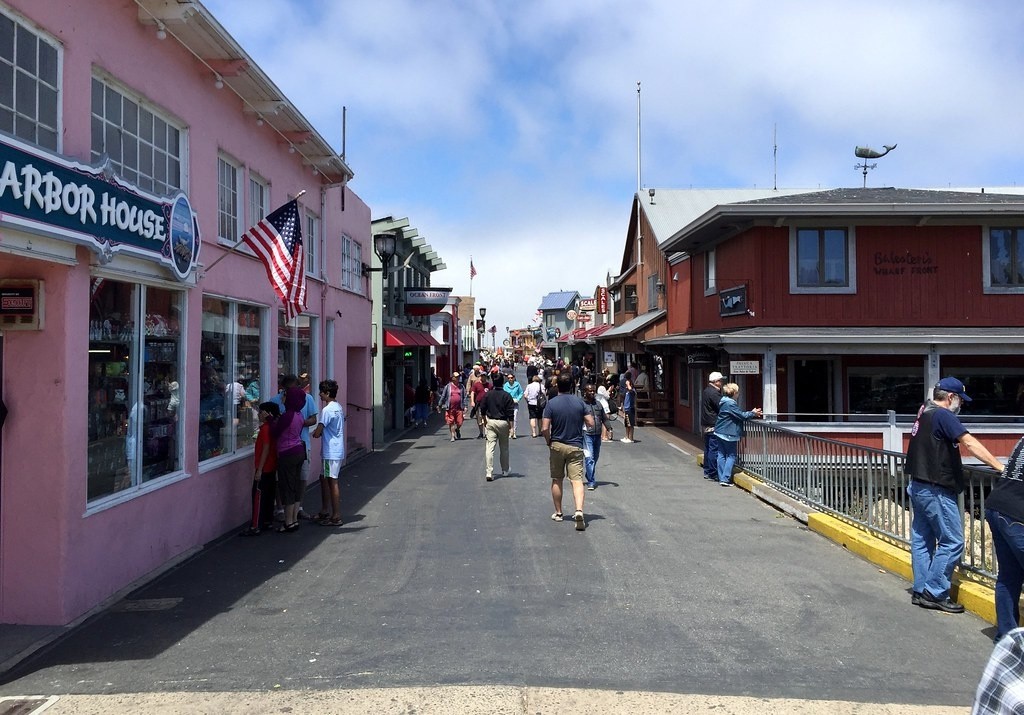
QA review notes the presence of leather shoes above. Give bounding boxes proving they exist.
[920,588,964,613]
[912,590,922,604]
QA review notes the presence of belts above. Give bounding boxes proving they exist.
[911,475,945,488]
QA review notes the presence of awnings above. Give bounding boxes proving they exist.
[384,328,441,346]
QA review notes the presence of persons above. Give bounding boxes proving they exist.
[985,436,1024,645]
[713,382,762,487]
[312,380,346,526]
[904,377,1004,614]
[541,373,596,531]
[244,375,261,439]
[405,358,523,442]
[524,355,649,444]
[479,373,514,481]
[240,374,318,537]
[226,375,245,417]
[579,384,613,490]
[700,371,727,481]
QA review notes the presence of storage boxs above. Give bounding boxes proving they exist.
[589,372,601,384]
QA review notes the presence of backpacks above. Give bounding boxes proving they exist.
[536,382,546,409]
[608,399,619,421]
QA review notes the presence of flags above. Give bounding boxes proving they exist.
[471,263,477,279]
[241,198,308,325]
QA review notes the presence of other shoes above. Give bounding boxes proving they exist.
[588,483,594,490]
[720,480,735,487]
[623,438,634,443]
[503,467,511,477]
[485,471,494,481]
[450,437,455,442]
[620,437,627,441]
[455,428,461,439]
[708,475,719,481]
[704,474,709,478]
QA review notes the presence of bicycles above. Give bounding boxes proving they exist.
[585,371,619,421]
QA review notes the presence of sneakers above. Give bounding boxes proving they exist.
[573,512,586,530]
[551,512,563,521]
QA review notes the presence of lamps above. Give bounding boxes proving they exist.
[361,234,398,279]
[631,290,638,304]
[649,189,655,204]
[655,278,667,297]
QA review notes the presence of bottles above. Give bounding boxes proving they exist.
[148,342,176,362]
[89,320,102,340]
[147,398,169,419]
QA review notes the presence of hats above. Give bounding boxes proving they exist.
[709,371,727,381]
[480,372,487,375]
[934,376,972,401]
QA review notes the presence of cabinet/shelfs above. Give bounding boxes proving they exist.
[88,335,310,500]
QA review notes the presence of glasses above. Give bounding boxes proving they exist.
[507,377,514,379]
[454,374,462,378]
